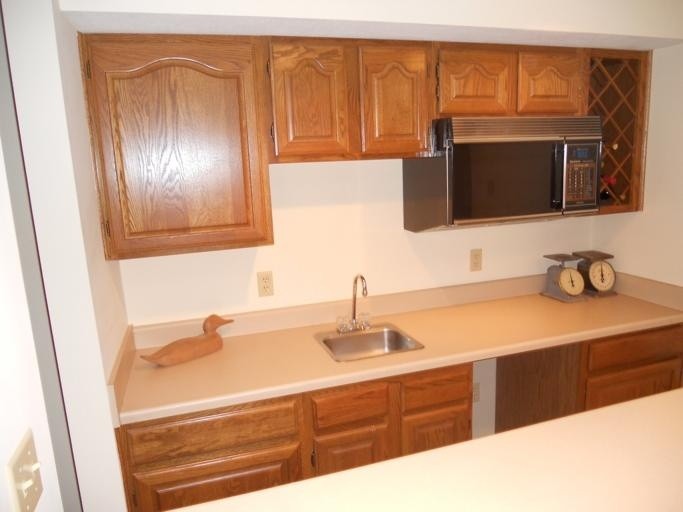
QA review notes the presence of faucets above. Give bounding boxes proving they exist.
[351,274,367,320]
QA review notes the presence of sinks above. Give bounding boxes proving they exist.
[315,322,425,362]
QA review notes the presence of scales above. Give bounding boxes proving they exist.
[539,249,617,304]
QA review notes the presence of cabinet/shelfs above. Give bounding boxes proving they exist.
[494,324,683,436]
[303,363,473,484]
[78,35,274,262]
[428,41,593,123]
[119,394,313,512]
[584,47,653,215]
[269,37,431,162]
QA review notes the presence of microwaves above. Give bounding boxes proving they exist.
[402,117,603,233]
